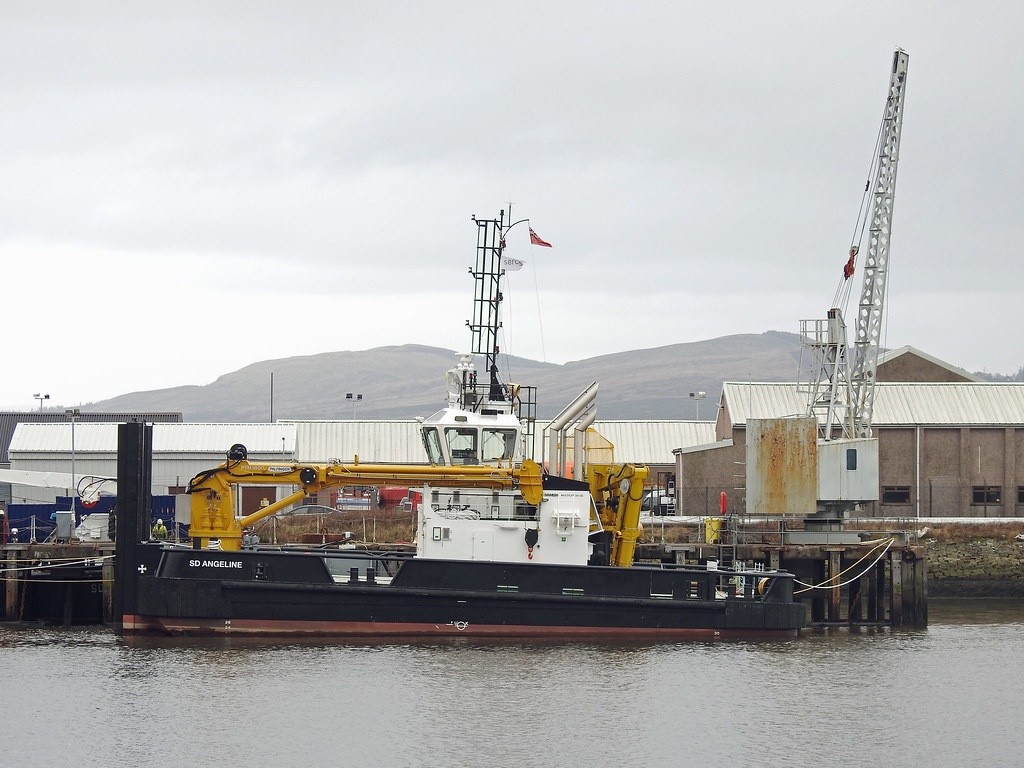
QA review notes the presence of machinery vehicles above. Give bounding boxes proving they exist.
[820,44,910,523]
[558,425,650,567]
[181,445,545,556]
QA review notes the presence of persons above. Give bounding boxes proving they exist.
[152,519,167,540]
[527,502,536,521]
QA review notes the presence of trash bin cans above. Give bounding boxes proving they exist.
[705,517,721,544]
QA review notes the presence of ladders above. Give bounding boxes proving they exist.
[719,515,737,598]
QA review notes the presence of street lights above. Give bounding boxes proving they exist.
[64,408,81,539]
[33,392,50,412]
[344,392,363,421]
[688,391,707,420]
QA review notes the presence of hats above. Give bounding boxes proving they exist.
[157,519,163,524]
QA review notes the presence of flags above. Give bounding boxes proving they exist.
[529,227,552,247]
[501,255,525,271]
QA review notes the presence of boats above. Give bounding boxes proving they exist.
[119,205,814,644]
